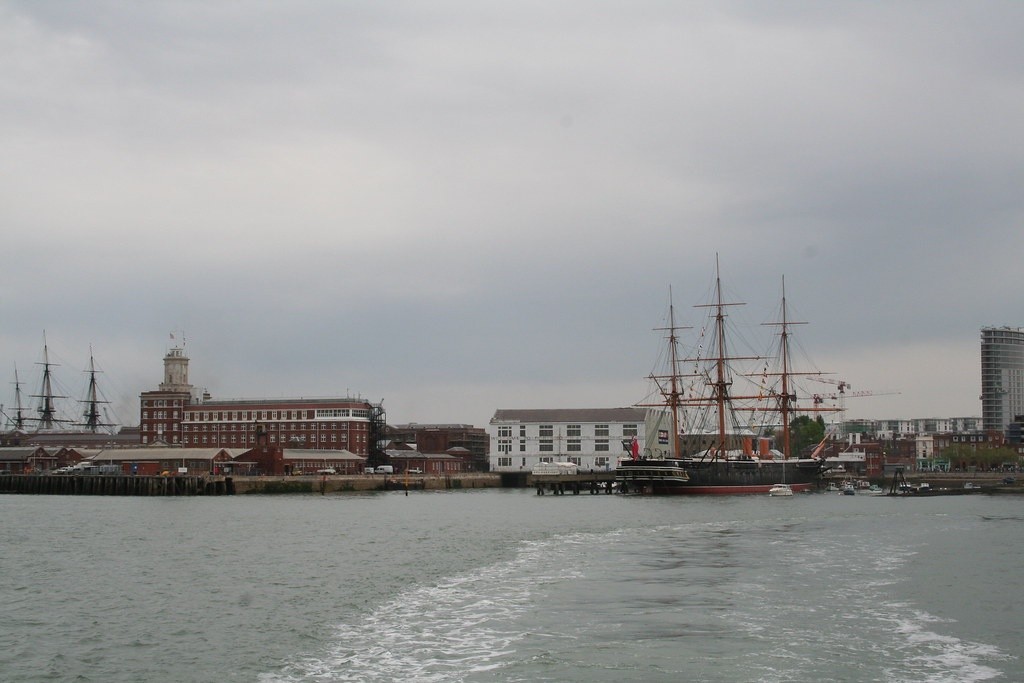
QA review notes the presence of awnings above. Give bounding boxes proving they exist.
[383,450,458,463]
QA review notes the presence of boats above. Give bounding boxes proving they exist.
[769,484,794,497]
[828,478,933,495]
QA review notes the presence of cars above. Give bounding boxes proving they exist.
[54,466,82,475]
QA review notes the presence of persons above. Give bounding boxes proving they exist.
[130,459,375,478]
[916,463,1024,472]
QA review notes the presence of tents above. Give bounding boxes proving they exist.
[533,462,578,475]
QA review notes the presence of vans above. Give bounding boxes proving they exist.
[375,465,393,474]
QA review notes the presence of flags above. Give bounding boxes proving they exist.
[749,361,771,430]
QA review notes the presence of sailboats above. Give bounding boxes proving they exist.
[611,252,846,497]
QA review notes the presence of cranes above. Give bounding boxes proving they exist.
[806,375,903,402]
